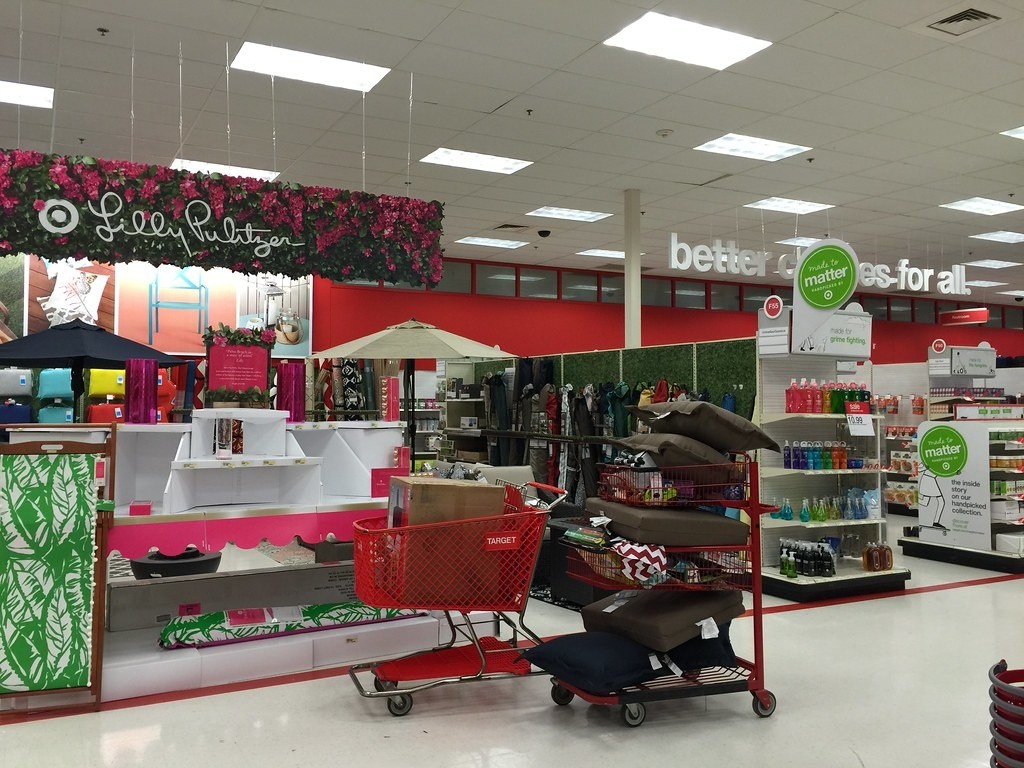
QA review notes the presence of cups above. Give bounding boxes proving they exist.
[215,418,232,460]
[864,456,869,469]
[868,458,879,468]
[848,459,856,468]
[856,458,864,468]
[248,318,262,326]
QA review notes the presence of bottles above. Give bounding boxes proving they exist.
[863,541,893,571]
[793,440,857,469]
[783,440,791,468]
[784,377,871,414]
[930,386,1001,397]
[928,404,949,414]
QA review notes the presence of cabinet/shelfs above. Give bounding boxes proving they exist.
[6,420,438,709]
[551,452,777,727]
[399,336,912,603]
[901,420,1024,575]
[872,361,1006,517]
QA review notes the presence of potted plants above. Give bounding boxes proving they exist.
[209,386,271,409]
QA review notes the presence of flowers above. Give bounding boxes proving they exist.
[202,324,276,349]
[0,147,448,287]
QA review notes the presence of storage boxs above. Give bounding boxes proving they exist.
[439,610,502,645]
[995,532,1024,554]
[383,476,505,603]
[460,417,486,429]
[990,480,1024,520]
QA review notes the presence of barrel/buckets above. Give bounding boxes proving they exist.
[276,362,305,423]
[124,357,158,424]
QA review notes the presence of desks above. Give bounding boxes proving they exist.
[240,314,309,358]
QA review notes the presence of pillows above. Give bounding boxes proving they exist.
[516,620,742,696]
[610,433,747,494]
[34,256,112,328]
[624,398,781,456]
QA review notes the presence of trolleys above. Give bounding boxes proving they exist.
[531,449,775,727]
[349,482,568,718]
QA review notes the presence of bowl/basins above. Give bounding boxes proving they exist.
[273,325,301,345]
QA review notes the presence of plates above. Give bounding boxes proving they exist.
[246,325,265,331]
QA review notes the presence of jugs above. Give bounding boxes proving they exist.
[277,308,303,335]
